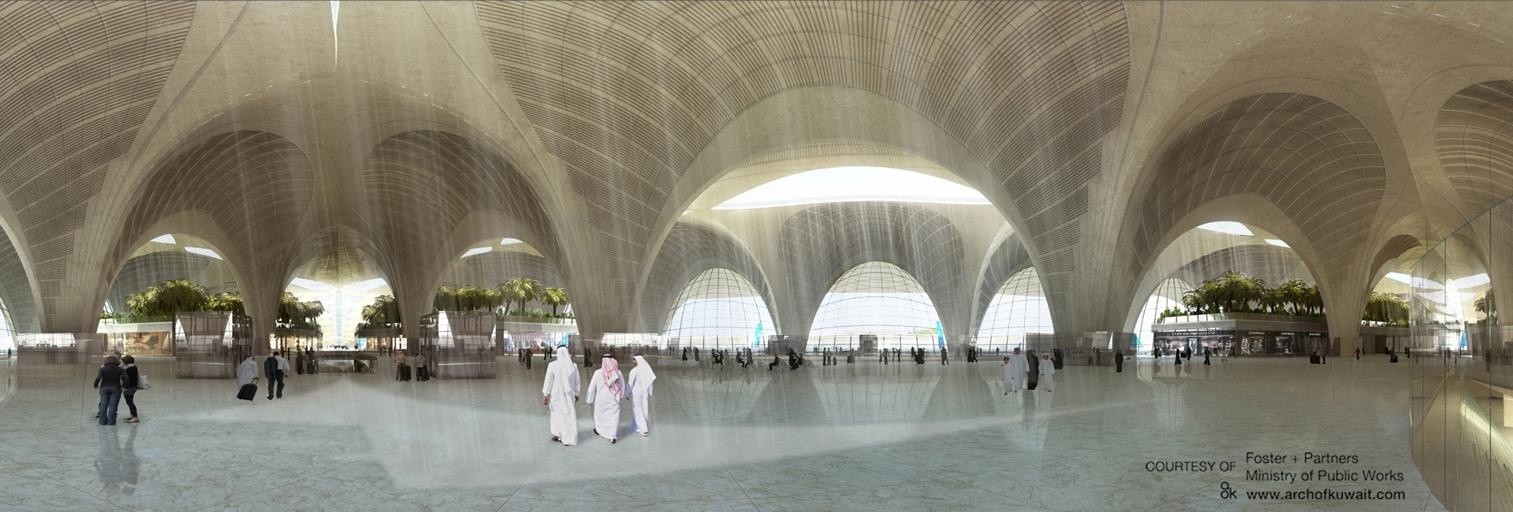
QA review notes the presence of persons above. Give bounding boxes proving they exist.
[519,344,657,445]
[1114,348,1123,373]
[879,347,925,364]
[1316,345,1320,364]
[768,346,856,370]
[387,346,430,382]
[93,350,140,425]
[1096,347,1101,366]
[235,344,319,399]
[1150,341,1224,366]
[1384,345,1410,363]
[1355,347,1360,359]
[966,345,1064,397]
[940,346,949,365]
[681,345,753,368]
[8,348,12,359]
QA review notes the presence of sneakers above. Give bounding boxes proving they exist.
[122,415,140,424]
[551,428,650,446]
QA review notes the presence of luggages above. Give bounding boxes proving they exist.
[237,376,259,402]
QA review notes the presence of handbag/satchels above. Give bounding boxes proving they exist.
[135,366,152,390]
